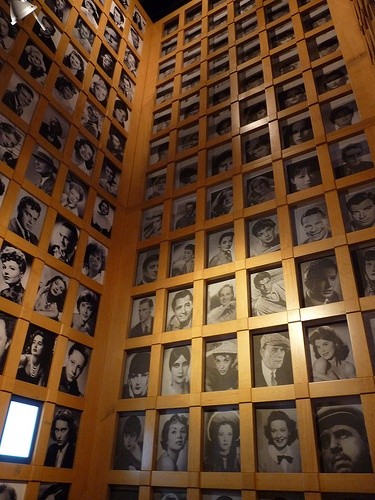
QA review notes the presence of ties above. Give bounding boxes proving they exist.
[55,449,63,467]
[25,230,29,241]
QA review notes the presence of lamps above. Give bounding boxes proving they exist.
[8,0,47,32]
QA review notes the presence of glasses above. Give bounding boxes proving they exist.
[320,431,366,447]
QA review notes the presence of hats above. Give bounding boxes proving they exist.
[205,341,237,358]
[109,130,124,147]
[115,99,128,121]
[32,145,59,175]
[260,332,290,349]
[315,405,366,435]
[208,411,240,442]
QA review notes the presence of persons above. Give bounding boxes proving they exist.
[203,410,241,472]
[153,0,355,172]
[155,414,189,471]
[1,4,143,399]
[43,409,78,468]
[257,411,302,472]
[0,483,68,500]
[315,405,374,473]
[122,143,375,397]
[112,415,143,470]
[162,493,178,500]
[216,495,233,500]
